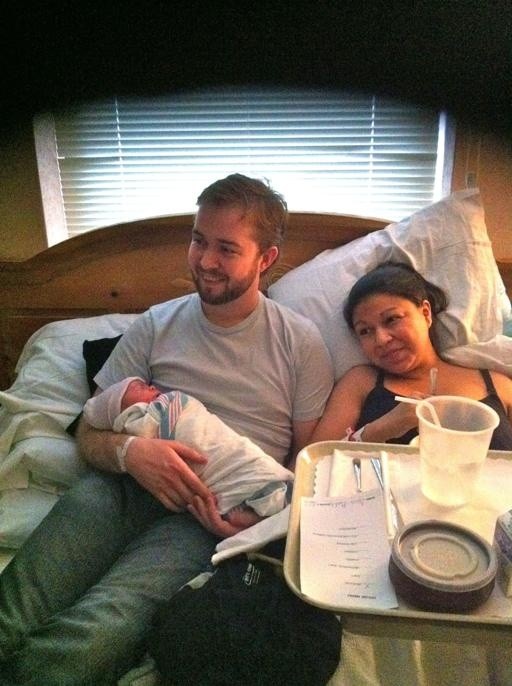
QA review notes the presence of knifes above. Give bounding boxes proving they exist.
[371,456,402,530]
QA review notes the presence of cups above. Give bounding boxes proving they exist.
[416,395,500,506]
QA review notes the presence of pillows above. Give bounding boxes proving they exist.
[1,477,67,553]
[269,187,512,386]
[0,309,138,486]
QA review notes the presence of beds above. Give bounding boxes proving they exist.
[0,205,512,685]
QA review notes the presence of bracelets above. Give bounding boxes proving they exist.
[116,435,136,473]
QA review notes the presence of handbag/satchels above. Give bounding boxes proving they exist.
[145,551,343,684]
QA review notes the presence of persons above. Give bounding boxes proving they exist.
[0,172,334,686]
[305,262,512,452]
[83,376,295,517]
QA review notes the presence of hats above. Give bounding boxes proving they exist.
[83,375,145,431]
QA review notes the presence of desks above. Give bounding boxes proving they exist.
[282,438,512,686]
